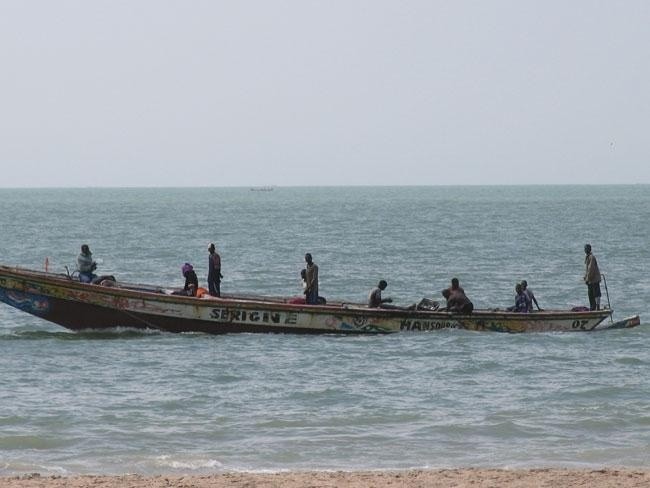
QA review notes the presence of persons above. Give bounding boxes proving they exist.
[583,244,602,311]
[206,243,223,297]
[180,261,198,295]
[512,283,530,313]
[505,279,545,312]
[366,278,416,310]
[75,243,99,284]
[302,251,321,305]
[447,278,466,294]
[440,287,474,315]
[299,269,309,304]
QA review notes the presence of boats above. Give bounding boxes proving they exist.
[0,265,641,335]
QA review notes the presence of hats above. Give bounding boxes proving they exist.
[207,243,215,249]
[182,262,194,273]
